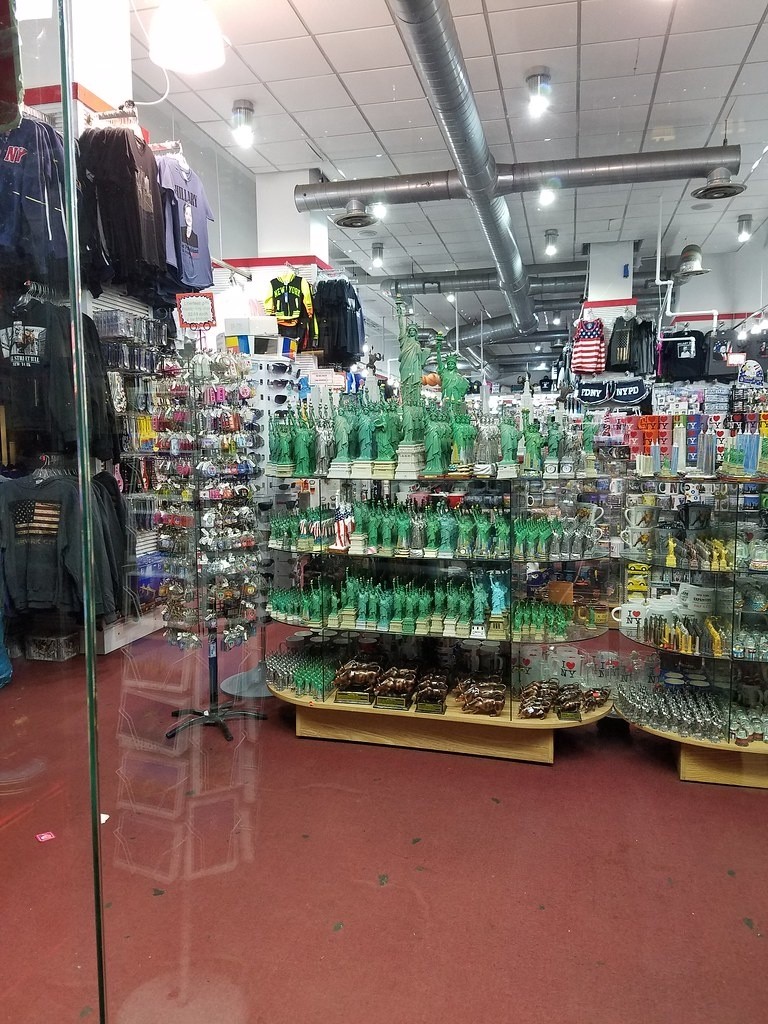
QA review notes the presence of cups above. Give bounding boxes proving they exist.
[396,481,502,513]
[278,630,504,680]
[511,479,768,708]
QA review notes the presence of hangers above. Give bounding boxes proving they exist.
[317,269,350,286]
[30,452,80,485]
[21,104,56,130]
[88,107,149,141]
[151,140,192,170]
[609,306,643,325]
[9,280,71,311]
[573,308,605,327]
[614,370,643,384]
[279,261,297,278]
[580,372,608,385]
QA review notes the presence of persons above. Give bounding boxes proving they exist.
[266,649,344,696]
[267,572,595,635]
[617,683,727,740]
[268,495,594,556]
[724,444,744,465]
[668,536,728,562]
[181,203,198,248]
[760,433,768,459]
[266,305,597,474]
[663,455,668,468]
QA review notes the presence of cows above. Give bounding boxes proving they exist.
[334,658,610,721]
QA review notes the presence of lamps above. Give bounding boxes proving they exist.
[524,65,552,119]
[447,292,454,302]
[552,310,560,325]
[731,304,768,340]
[544,229,560,256]
[372,243,383,268]
[231,99,255,150]
[737,214,752,242]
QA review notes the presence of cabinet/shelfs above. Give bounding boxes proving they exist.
[613,469,768,789]
[263,460,613,764]
